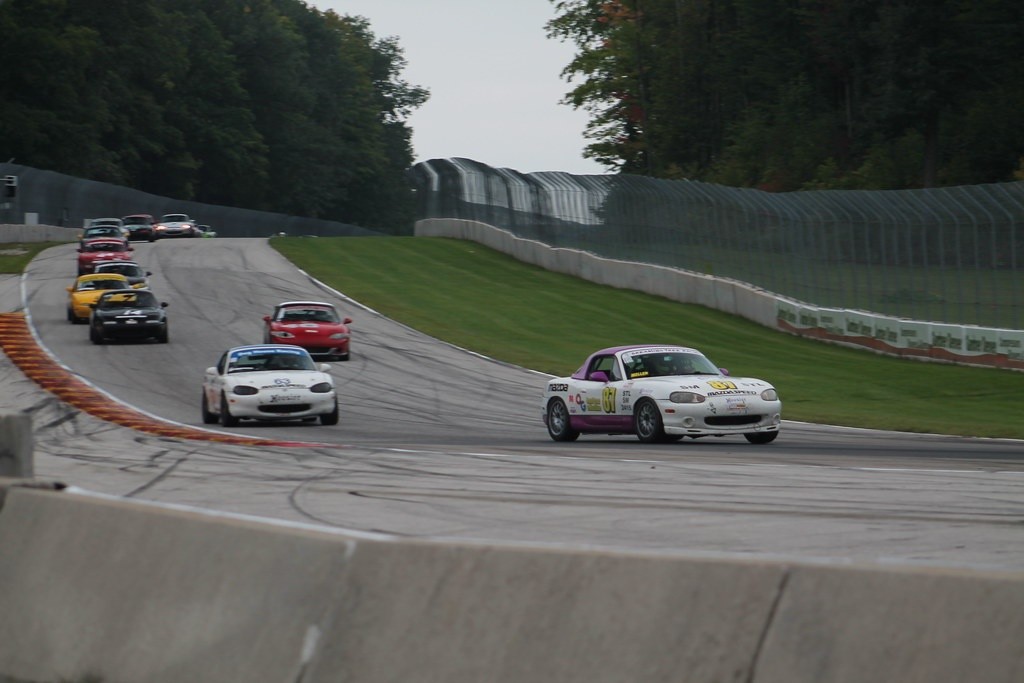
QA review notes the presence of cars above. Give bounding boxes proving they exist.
[91,263,152,292]
[121,215,155,242]
[89,218,130,238]
[539,343,783,442]
[78,238,133,274]
[154,212,219,240]
[88,289,171,343]
[67,274,138,322]
[260,302,354,359]
[201,345,339,426]
[81,226,131,250]
